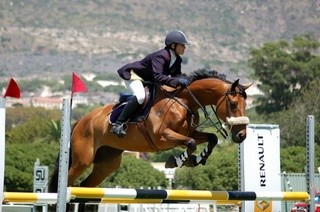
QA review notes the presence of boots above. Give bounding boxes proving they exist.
[108,95,141,137]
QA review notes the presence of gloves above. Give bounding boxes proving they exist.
[179,78,189,88]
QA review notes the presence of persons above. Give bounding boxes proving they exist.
[108,30,191,138]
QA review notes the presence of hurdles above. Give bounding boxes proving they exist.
[0,98,316,212]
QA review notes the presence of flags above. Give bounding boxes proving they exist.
[3,79,21,100]
[71,72,87,94]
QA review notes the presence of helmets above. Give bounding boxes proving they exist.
[165,31,189,46]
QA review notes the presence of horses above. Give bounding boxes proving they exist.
[36,68,254,212]
